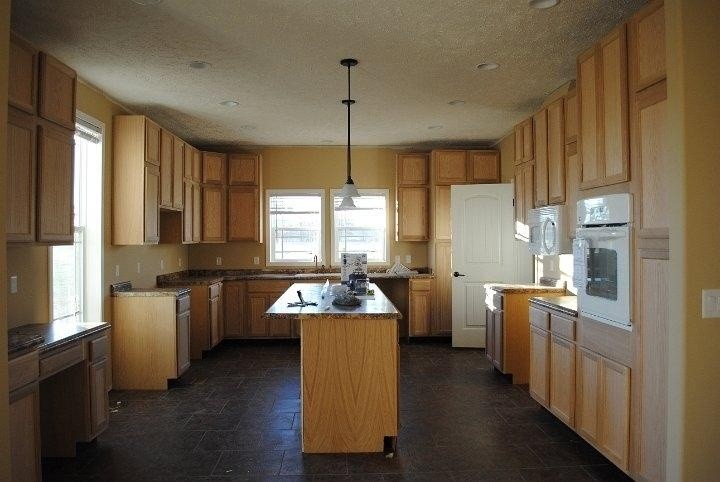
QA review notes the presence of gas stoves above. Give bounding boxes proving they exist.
[8,332,45,352]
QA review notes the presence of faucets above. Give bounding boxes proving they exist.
[314,255,317,272]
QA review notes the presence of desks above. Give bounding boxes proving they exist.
[265,283,403,455]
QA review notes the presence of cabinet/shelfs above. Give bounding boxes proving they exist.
[190,280,300,360]
[484,292,503,371]
[112,114,266,246]
[177,294,191,374]
[395,152,428,243]
[530,304,576,428]
[410,277,432,338]
[9,315,111,482]
[576,347,631,469]
[512,23,630,229]
[7,39,77,243]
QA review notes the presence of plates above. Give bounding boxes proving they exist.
[331,298,363,307]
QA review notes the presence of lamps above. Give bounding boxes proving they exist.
[340,59,361,197]
[338,100,356,209]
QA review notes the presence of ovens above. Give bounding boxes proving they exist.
[573,192,636,332]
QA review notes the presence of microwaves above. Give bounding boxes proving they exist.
[527,204,569,257]
